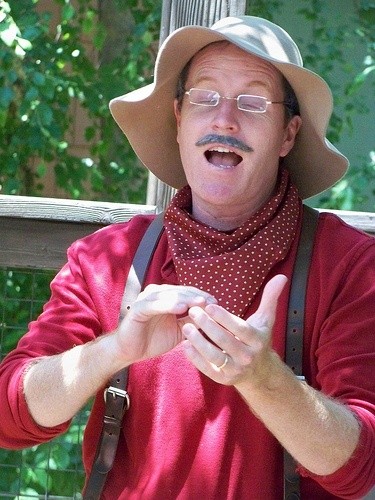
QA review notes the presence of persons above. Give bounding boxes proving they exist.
[0,14,375,500]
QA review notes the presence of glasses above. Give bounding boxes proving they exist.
[178,80,293,113]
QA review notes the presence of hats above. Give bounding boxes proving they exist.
[109,15,349,201]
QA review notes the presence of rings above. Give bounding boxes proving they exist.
[217,351,230,370]
